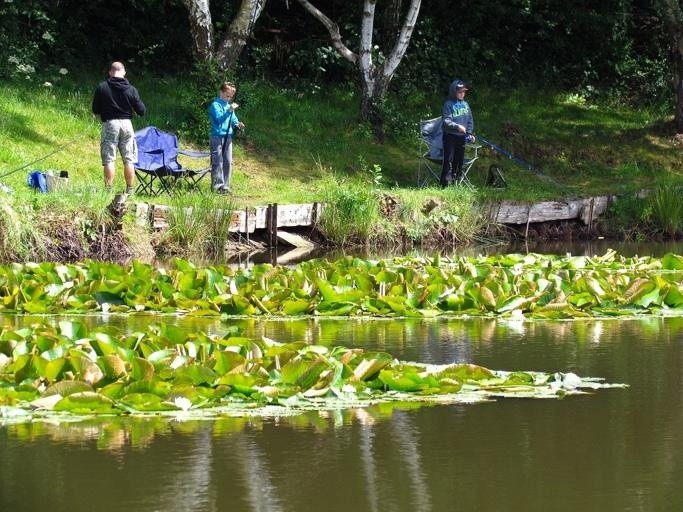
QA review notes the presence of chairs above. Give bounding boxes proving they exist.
[418,117,482,191]
[133,125,212,193]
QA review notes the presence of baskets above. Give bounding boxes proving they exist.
[47,170,71,192]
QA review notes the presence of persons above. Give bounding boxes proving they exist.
[442,79,474,189]
[92,61,146,196]
[208,81,245,194]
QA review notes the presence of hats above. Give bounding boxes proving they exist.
[456,84,468,92]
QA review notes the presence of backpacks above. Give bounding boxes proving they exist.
[486,163,507,188]
[27,172,46,193]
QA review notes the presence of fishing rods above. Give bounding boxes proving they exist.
[222,1,259,154]
[464,130,576,200]
[0,119,97,178]
